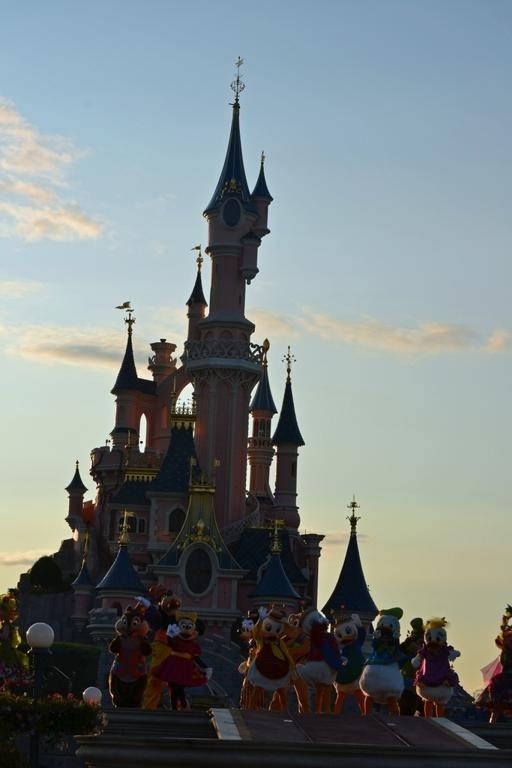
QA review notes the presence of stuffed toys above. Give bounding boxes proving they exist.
[151,611,214,713]
[107,596,152,710]
[133,589,183,710]
[231,598,461,722]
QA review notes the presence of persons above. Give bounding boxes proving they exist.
[470,603,511,723]
[0,593,32,670]
